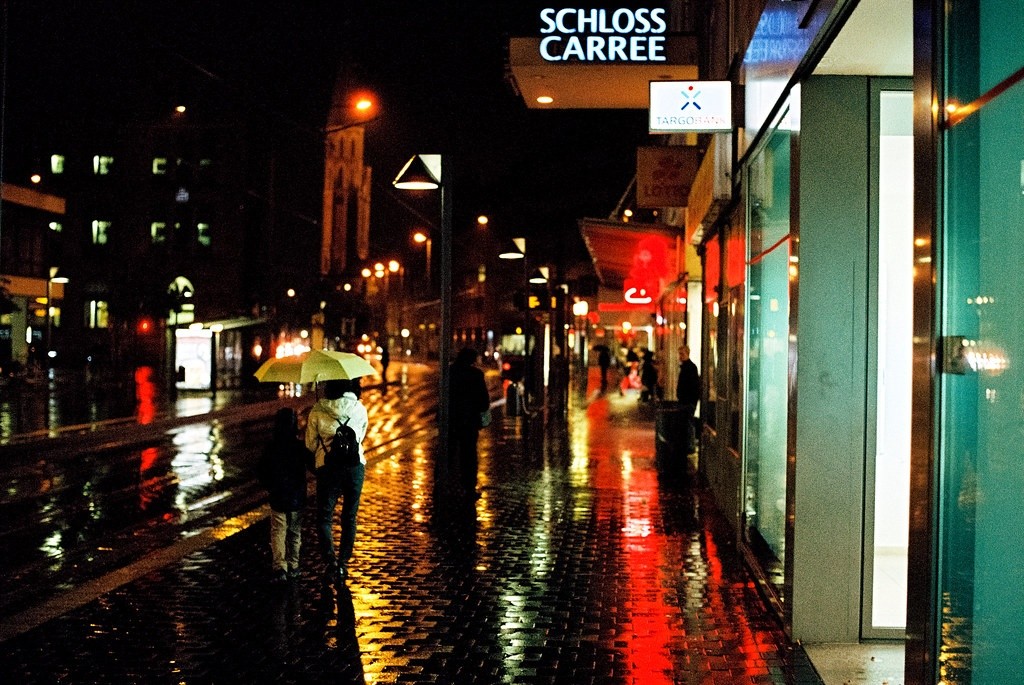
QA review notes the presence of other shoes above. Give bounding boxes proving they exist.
[289,567,301,578]
[273,571,285,580]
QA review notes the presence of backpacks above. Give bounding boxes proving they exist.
[319,418,367,469]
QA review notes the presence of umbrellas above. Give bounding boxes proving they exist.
[253,351,378,402]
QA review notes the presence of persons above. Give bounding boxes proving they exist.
[555,342,664,413]
[305,378,368,575]
[677,346,700,440]
[438,348,490,486]
[263,407,315,579]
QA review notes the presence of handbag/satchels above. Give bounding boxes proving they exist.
[480,410,493,427]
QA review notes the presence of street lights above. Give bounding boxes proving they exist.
[498,234,532,437]
[393,147,458,466]
[43,275,70,432]
[529,265,555,397]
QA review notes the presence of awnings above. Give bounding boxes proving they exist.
[580,216,679,289]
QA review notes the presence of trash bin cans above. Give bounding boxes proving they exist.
[654,398,697,456]
